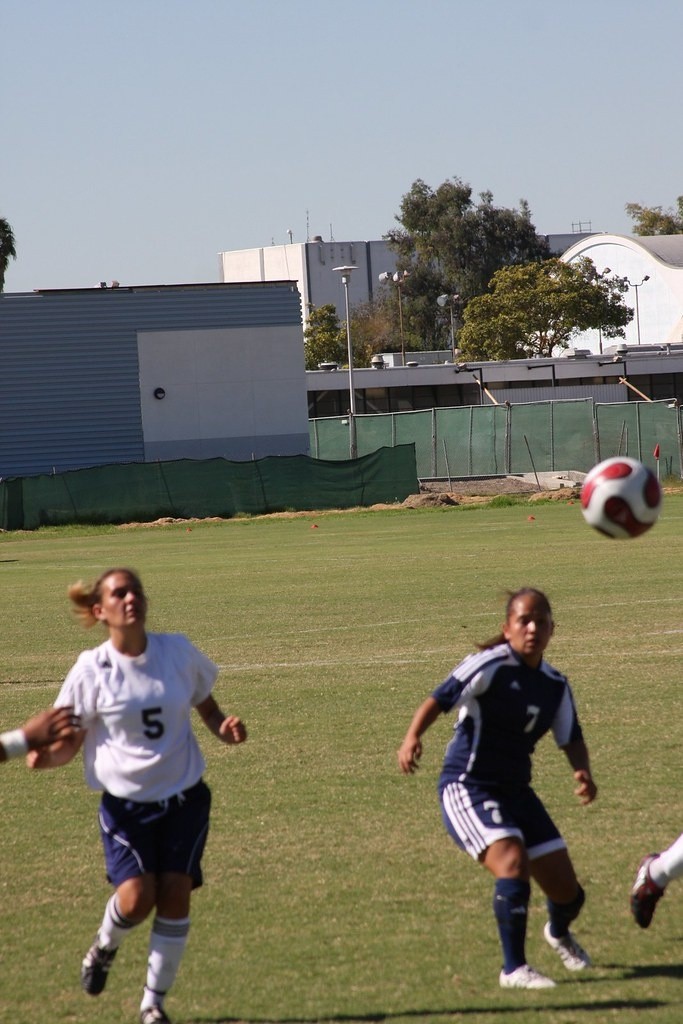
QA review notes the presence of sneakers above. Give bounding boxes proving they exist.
[629,853,665,928]
[543,921,591,972]
[81,927,118,996]
[140,1002,170,1024]
[499,964,557,990]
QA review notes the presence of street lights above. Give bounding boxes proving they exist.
[332,265,362,415]
[621,275,650,346]
[436,293,462,363]
[378,270,409,367]
[586,267,611,354]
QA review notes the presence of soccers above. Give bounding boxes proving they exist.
[579,455,663,542]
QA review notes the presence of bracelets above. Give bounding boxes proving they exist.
[0,729,27,762]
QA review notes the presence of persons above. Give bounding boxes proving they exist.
[399,587,598,987]
[0,705,81,764]
[630,832,683,929]
[28,569,247,1024]
